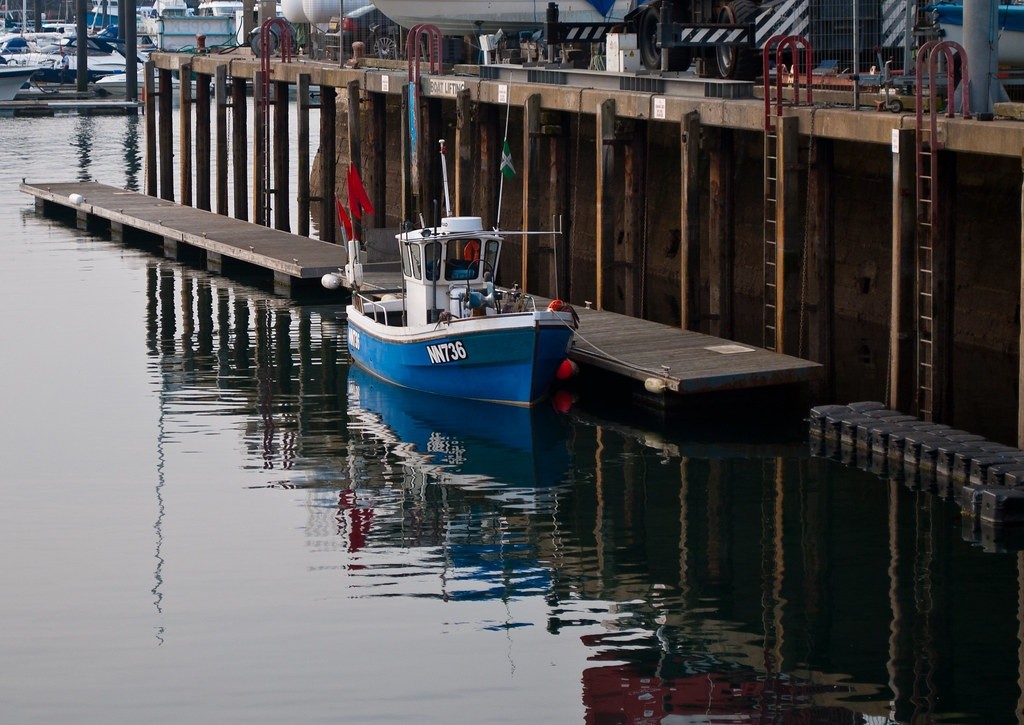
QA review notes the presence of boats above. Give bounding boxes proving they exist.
[345,214,577,409]
[0,0,243,98]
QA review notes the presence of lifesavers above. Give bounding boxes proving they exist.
[464,240,480,262]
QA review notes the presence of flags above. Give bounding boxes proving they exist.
[335,194,358,240]
[500,137,517,181]
[346,161,375,218]
